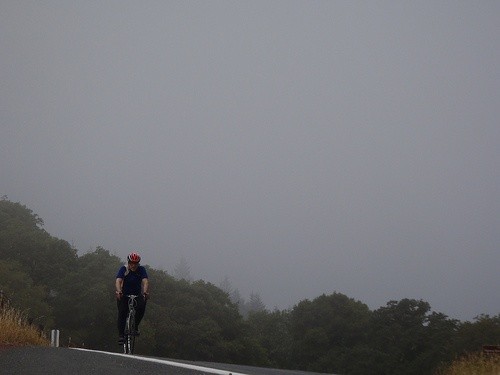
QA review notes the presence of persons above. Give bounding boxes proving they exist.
[116,253,150,345]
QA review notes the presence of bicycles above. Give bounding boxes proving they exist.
[117,292,149,354]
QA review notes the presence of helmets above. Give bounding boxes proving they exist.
[127,252,140,263]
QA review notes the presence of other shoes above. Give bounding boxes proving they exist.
[118,335,124,345]
